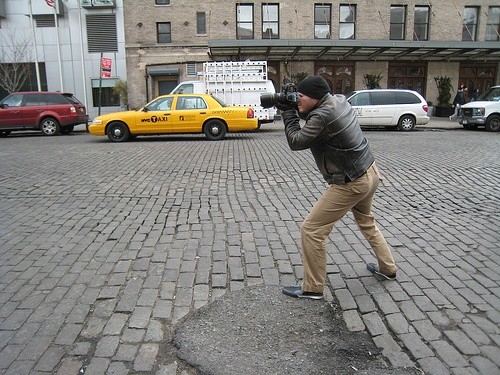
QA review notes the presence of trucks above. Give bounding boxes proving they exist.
[152,60,282,133]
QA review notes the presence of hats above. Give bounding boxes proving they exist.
[297,75,331,100]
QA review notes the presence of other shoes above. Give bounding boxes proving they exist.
[449,116,452,121]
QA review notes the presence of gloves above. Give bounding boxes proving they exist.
[275,100,294,112]
[283,83,297,93]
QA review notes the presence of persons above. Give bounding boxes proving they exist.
[449,86,469,120]
[167,99,172,108]
[282,76,396,299]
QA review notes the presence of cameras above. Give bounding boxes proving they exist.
[259,83,298,109]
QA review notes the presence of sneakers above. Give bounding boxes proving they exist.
[367,263,396,281]
[282,285,323,300]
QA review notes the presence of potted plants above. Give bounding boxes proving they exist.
[434,76,455,117]
[426,100,434,117]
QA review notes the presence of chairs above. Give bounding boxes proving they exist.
[177,98,187,110]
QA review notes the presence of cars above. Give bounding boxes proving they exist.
[87,92,258,143]
[456,85,500,132]
[345,88,431,132]
[0,91,89,138]
